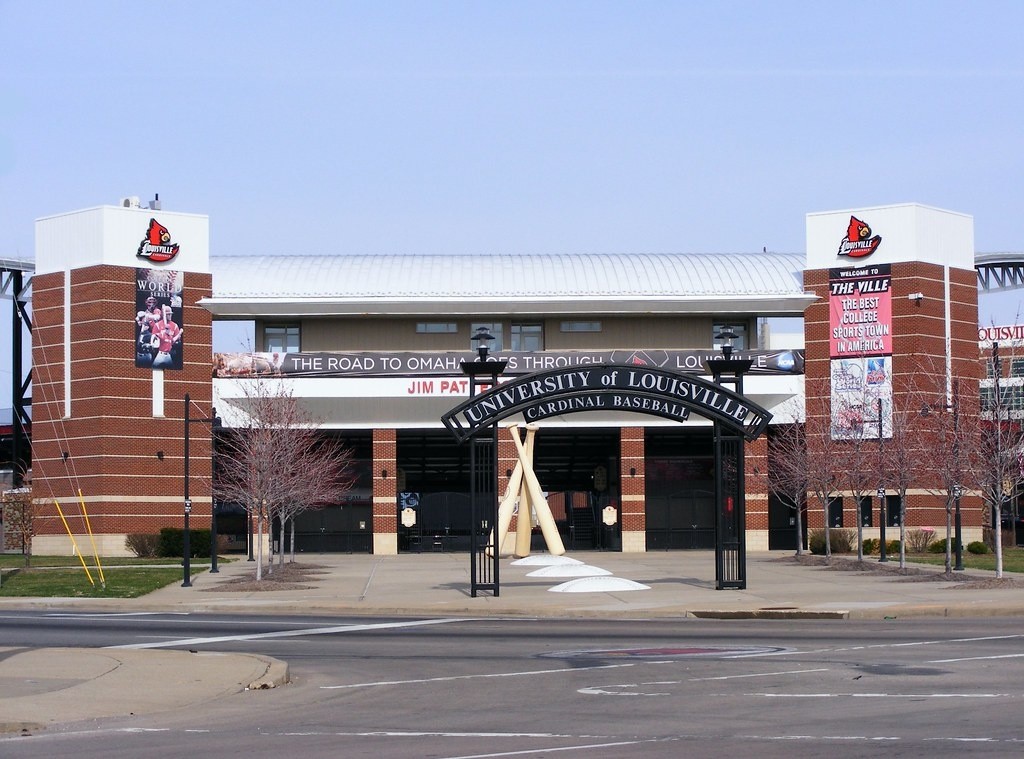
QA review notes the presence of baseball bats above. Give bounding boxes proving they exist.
[162,303,169,343]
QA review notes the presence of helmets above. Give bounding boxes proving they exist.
[145,297,157,312]
[160,306,173,315]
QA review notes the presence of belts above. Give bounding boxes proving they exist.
[161,352,170,355]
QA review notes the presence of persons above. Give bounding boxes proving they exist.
[135,297,161,360]
[150,306,181,366]
[405,493,418,508]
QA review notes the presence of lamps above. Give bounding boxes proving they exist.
[754,467,760,473]
[507,469,511,477]
[157,451,164,460]
[631,468,636,475]
[382,470,387,477]
[61,451,69,458]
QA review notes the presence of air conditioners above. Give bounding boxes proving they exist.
[120,197,140,208]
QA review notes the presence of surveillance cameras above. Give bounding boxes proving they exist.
[918,294,923,300]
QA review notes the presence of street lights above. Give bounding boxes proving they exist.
[848,418,889,562]
[922,401,964,571]
[182,418,223,587]
